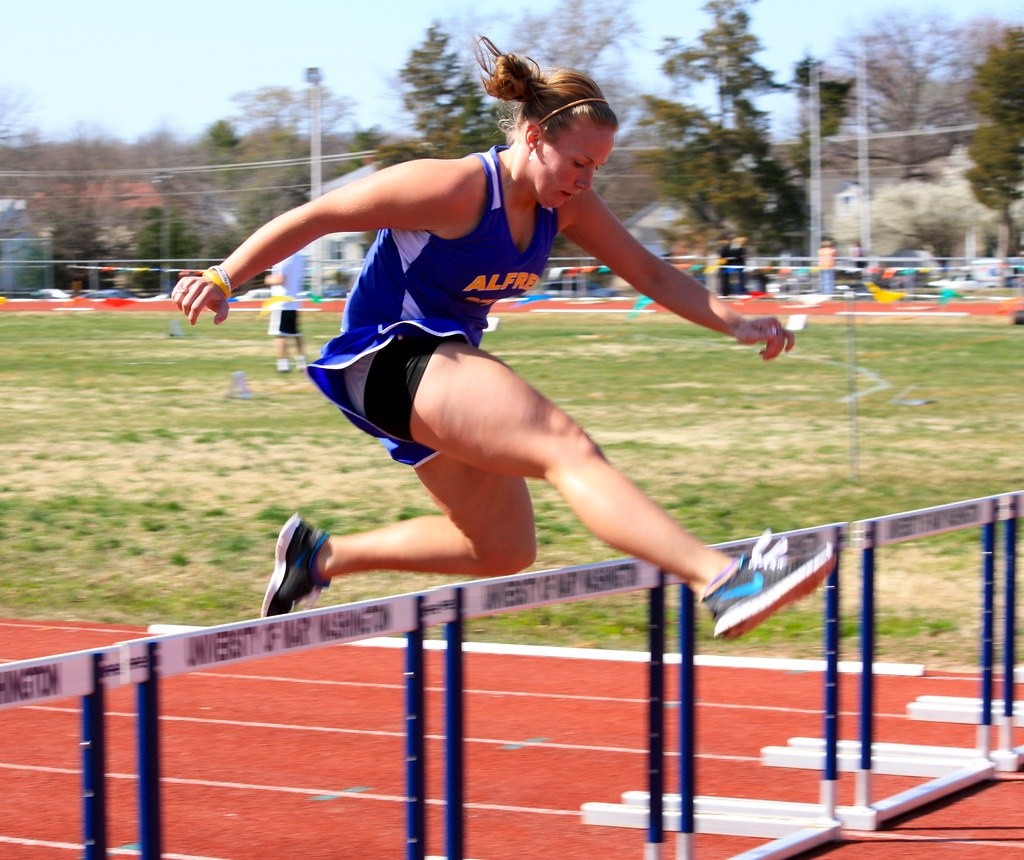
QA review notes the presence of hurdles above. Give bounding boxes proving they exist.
[0,490,1024,860]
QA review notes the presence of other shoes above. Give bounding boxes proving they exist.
[275,358,290,373]
[295,355,307,371]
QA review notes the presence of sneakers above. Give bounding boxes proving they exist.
[260,513,331,617]
[703,529,837,642]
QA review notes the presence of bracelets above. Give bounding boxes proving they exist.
[205,263,234,301]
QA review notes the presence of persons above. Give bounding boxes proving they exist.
[818,235,836,294]
[717,235,747,297]
[170,34,835,644]
[267,247,309,374]
[854,241,867,269]
